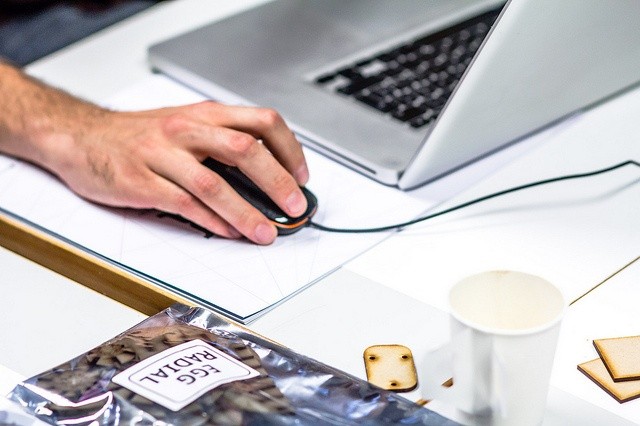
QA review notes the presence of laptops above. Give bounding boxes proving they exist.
[145,0,640,190]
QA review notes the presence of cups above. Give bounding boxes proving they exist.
[445,269,567,425]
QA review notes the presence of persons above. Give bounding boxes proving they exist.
[0,63,310,245]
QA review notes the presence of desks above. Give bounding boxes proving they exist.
[0,0,639,425]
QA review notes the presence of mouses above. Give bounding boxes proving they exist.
[198,157,318,235]
